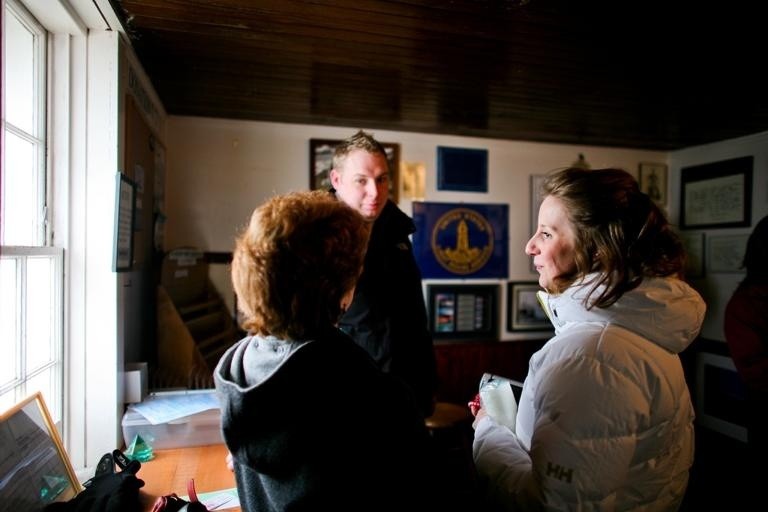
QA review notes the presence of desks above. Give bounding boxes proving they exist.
[116,442,242,509]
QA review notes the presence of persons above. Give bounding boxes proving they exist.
[212,185,466,510]
[720,213,767,457]
[468,165,711,511]
[321,127,440,424]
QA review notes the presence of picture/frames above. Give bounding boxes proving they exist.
[0,390,83,511]
[639,162,668,206]
[308,140,400,207]
[117,170,138,272]
[508,280,554,333]
